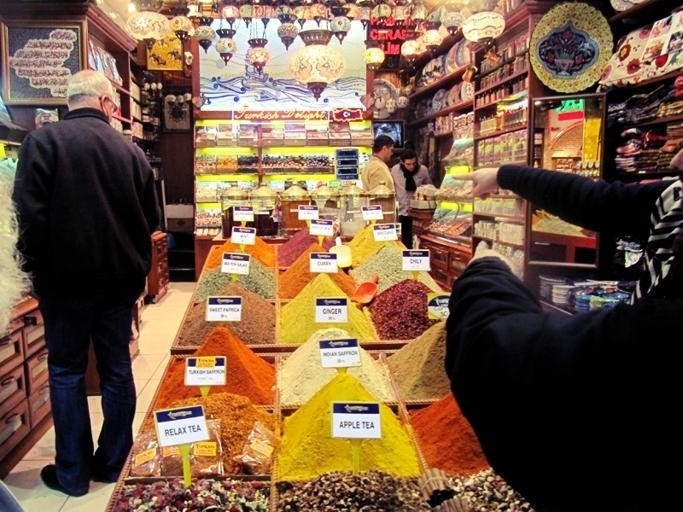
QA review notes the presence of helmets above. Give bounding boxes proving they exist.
[528,1,615,93]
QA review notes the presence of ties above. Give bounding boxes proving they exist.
[374,119,406,153]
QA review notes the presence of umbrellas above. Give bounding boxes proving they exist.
[41,465,88,496]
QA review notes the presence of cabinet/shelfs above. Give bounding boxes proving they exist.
[474,32,528,108]
[0,0,683,512]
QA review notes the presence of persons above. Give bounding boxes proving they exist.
[11,69,156,496]
[390,150,433,248]
[443,147,683,512]
[361,134,395,194]
